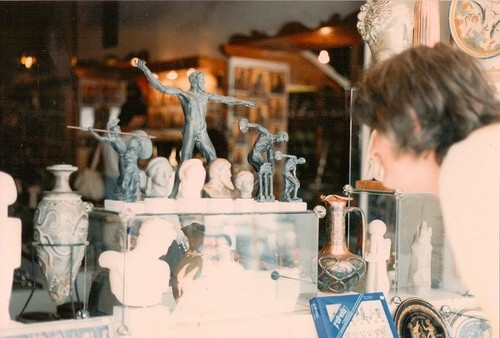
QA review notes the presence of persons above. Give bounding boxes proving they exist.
[118,81,149,132]
[350,41,500,337]
[1,112,47,211]
[93,92,109,133]
[356,1,416,68]
[87,56,308,337]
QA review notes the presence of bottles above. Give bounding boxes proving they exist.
[33,164,89,302]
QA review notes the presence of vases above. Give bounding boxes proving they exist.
[317,194,367,295]
[33,165,93,307]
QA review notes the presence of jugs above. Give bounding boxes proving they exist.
[318,194,366,293]
